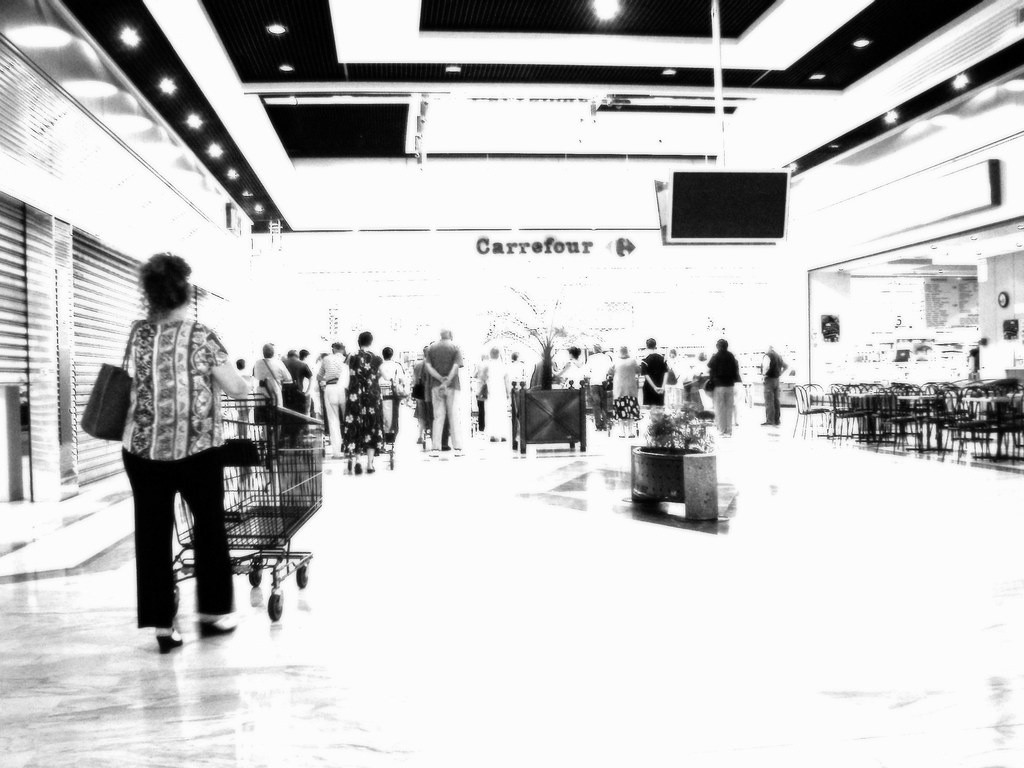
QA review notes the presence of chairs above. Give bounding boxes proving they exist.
[793,378,1024,466]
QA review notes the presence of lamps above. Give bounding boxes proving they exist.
[416,114,427,134]
[420,99,429,117]
[590,100,597,117]
[414,135,425,168]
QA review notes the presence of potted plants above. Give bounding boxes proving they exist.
[631,404,715,503]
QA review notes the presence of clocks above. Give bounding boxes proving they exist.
[998,292,1009,307]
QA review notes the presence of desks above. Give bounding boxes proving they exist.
[961,394,1024,462]
[847,393,910,444]
[897,395,959,453]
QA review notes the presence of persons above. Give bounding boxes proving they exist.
[759,343,788,426]
[119,251,250,654]
[234,330,467,475]
[472,346,527,442]
[708,339,737,438]
[559,338,709,440]
[530,351,559,389]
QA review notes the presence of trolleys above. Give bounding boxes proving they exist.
[598,372,639,437]
[172,378,326,622]
[348,383,399,473]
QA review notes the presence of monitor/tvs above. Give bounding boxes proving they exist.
[654,167,793,247]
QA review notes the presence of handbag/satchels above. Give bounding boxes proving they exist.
[703,378,715,392]
[81,319,150,442]
[393,361,410,398]
[412,363,425,399]
[335,353,350,394]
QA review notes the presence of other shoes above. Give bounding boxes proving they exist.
[155,627,183,654]
[199,618,238,636]
[354,462,362,474]
[367,466,375,472]
